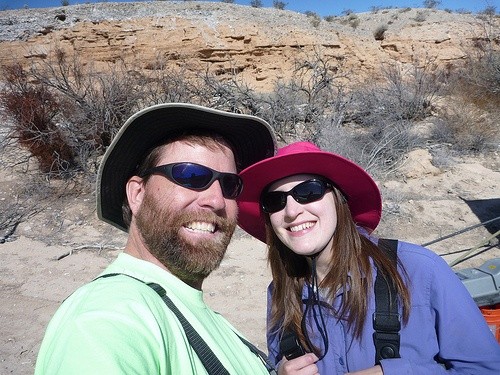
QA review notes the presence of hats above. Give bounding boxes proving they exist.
[96,102,277,234]
[236,142,382,245]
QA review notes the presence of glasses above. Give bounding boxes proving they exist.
[260,178,332,214]
[133,162,244,199]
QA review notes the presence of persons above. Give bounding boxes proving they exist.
[233,142,500,375]
[32,103,279,375]
